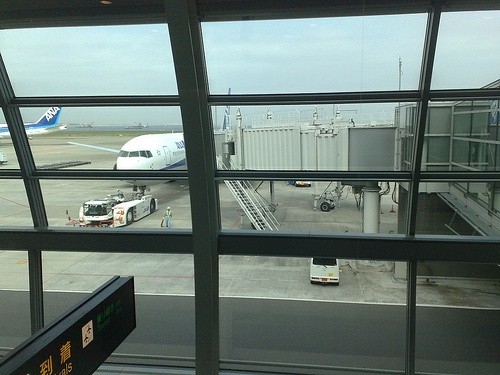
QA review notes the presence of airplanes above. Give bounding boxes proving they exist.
[66,87,233,185]
[0,106,68,141]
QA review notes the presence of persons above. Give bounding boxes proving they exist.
[163,206,172,228]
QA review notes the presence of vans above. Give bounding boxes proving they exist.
[309,257,341,285]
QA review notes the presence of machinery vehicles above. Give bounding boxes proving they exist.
[77,185,159,229]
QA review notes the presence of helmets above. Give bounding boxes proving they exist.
[167,207,171,209]
